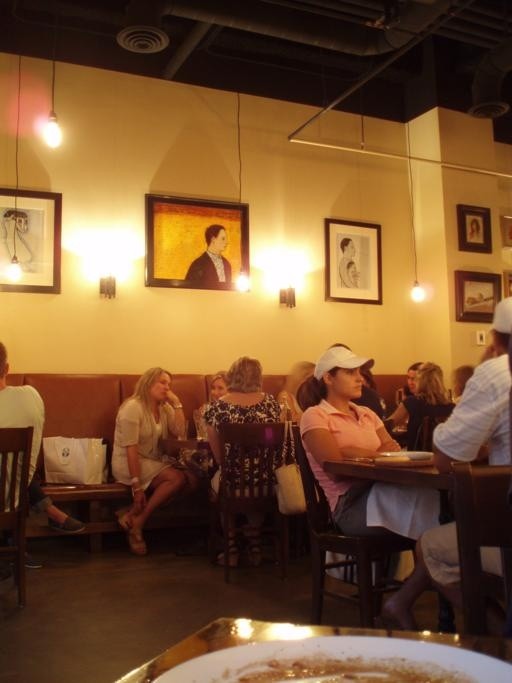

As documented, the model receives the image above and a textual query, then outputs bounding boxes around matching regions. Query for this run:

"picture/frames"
[144,192,251,293]
[323,217,383,306]
[0,188,64,296]
[498,215,512,251]
[501,269,512,298]
[455,203,493,254]
[454,270,502,324]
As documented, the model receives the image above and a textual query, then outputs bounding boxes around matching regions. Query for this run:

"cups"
[177,420,189,441]
[193,410,207,439]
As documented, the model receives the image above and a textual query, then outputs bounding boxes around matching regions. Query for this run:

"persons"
[418,296,512,635]
[337,237,359,289]
[111,367,199,555]
[202,355,280,567]
[23,482,86,569]
[299,347,453,632]
[185,224,232,285]
[0,342,45,511]
[469,219,482,243]
[276,343,473,451]
[194,369,226,441]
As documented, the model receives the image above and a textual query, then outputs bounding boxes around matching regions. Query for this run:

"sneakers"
[47,514,88,535]
[9,556,48,570]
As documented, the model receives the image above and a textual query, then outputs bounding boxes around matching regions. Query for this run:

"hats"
[489,294,512,335]
[312,345,376,381]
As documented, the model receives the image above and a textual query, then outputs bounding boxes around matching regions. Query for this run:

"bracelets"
[129,488,143,495]
[173,403,183,409]
[130,476,139,484]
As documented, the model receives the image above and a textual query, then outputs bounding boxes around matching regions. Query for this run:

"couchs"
[0,372,429,556]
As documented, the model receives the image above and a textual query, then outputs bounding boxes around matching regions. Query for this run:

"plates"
[147,634,512,682]
[380,451,433,460]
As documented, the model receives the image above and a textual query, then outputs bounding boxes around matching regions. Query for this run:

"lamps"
[89,247,123,301]
[38,54,65,150]
[230,89,252,293]
[405,117,427,307]
[4,52,25,286]
[273,266,305,310]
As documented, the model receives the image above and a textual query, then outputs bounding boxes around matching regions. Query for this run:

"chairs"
[206,421,291,587]
[421,415,448,452]
[0,426,35,610]
[291,419,415,628]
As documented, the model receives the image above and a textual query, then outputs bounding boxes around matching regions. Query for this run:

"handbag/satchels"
[41,435,109,485]
[274,462,322,517]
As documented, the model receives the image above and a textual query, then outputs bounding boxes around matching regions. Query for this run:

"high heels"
[213,543,241,567]
[128,527,148,555]
[114,505,134,530]
[372,614,433,632]
[244,545,263,569]
